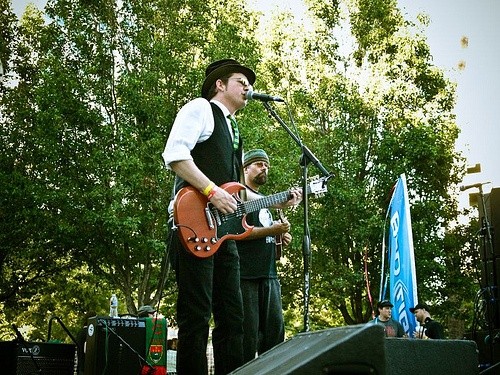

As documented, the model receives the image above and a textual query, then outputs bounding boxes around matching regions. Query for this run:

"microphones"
[460,182,490,190]
[246,91,285,102]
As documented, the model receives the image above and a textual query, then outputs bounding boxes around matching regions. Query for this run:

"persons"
[367,300,405,337]
[409,303,436,339]
[243,149,292,367]
[167,322,179,350]
[162,59,303,375]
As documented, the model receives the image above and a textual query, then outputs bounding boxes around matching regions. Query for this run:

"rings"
[298,197,301,199]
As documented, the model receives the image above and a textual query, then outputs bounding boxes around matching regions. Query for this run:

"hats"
[243,149,269,168]
[378,300,393,308]
[201,59,256,98]
[409,304,430,314]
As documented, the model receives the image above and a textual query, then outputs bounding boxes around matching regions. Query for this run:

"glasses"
[252,162,269,169]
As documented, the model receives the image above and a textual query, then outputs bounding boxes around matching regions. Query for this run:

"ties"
[227,114,239,153]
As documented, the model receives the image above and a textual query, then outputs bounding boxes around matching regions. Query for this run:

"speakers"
[84,316,167,375]
[228,187,500,375]
[0,340,76,375]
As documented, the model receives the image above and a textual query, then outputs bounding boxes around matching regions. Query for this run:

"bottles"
[109,293,118,318]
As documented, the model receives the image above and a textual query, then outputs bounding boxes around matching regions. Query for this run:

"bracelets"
[202,182,218,200]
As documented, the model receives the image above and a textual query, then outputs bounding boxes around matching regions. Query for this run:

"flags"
[390,174,418,338]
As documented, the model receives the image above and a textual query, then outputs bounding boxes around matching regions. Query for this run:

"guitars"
[274,208,285,259]
[173,173,329,259]
